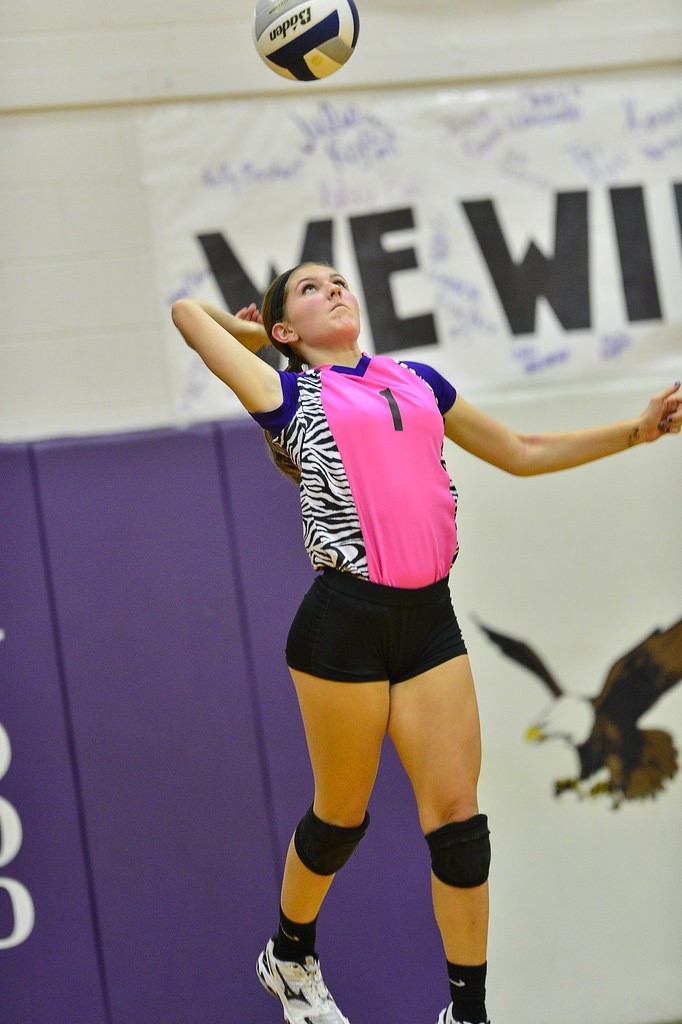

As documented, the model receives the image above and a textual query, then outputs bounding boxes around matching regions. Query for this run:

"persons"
[169,257,681,1024]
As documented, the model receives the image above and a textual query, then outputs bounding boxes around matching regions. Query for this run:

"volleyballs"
[252,1,359,81]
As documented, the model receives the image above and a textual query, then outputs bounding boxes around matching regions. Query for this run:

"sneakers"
[254,934,351,1024]
[434,1000,491,1024]
